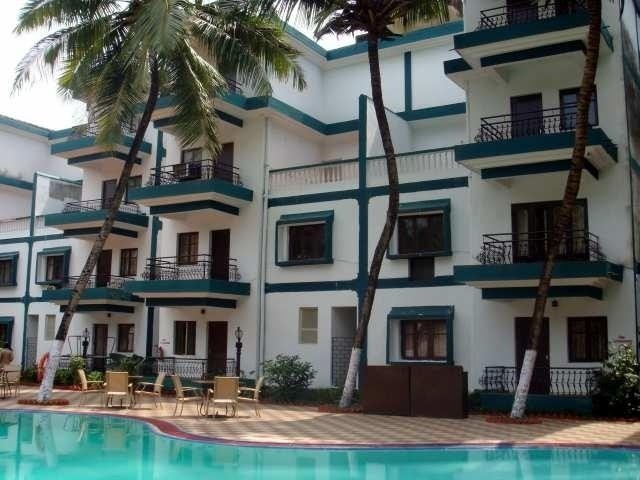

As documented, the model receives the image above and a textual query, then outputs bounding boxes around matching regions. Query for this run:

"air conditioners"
[173,163,192,179]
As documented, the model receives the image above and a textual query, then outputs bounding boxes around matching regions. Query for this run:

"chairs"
[0,364,22,398]
[77,368,168,410]
[171,372,265,420]
